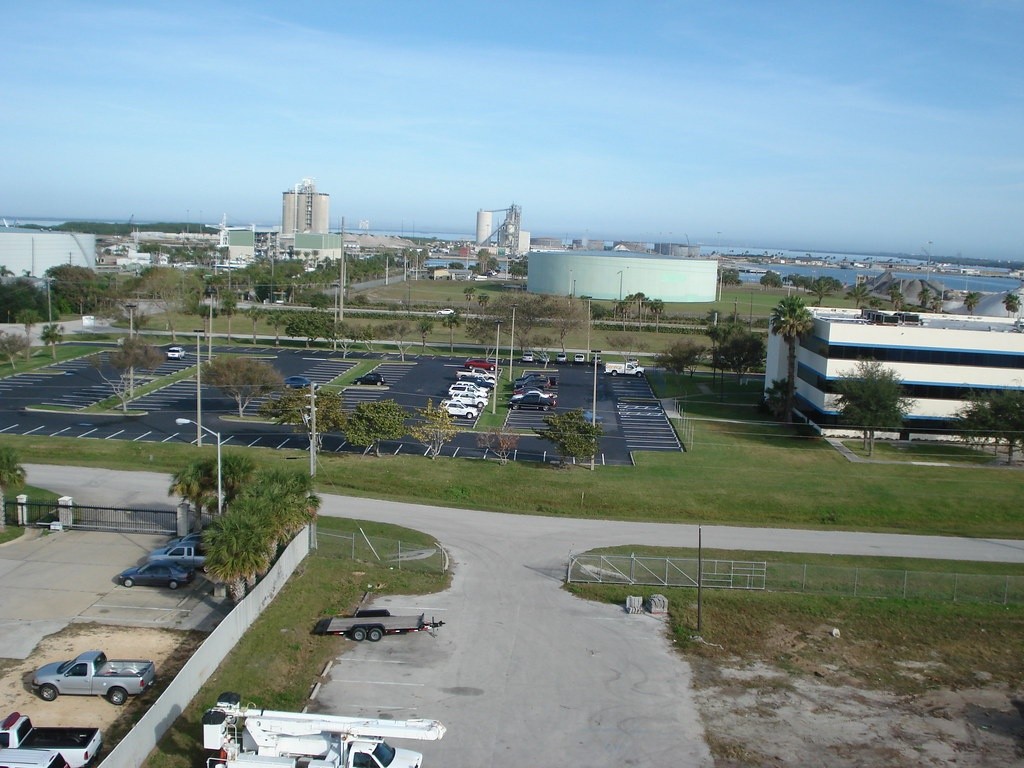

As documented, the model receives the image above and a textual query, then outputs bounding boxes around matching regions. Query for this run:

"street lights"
[176,418,222,515]
[194,330,205,447]
[509,304,519,381]
[586,296,592,362]
[590,350,602,470]
[207,291,216,360]
[126,304,136,397]
[492,319,503,414]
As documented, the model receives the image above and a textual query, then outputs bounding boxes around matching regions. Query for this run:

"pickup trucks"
[0,712,103,768]
[31,651,155,705]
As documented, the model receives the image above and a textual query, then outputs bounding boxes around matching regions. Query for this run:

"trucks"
[604,362,646,378]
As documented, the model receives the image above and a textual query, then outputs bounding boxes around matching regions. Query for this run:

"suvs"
[353,373,385,386]
[0,748,71,768]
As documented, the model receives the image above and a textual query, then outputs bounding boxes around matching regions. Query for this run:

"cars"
[284,376,319,390]
[438,358,495,420]
[507,374,556,410]
[555,352,568,364]
[118,531,207,590]
[167,346,185,360]
[573,353,585,363]
[437,308,456,315]
[591,356,602,366]
[540,354,549,362]
[521,352,536,363]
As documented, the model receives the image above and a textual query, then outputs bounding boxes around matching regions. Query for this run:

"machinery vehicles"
[202,691,447,768]
